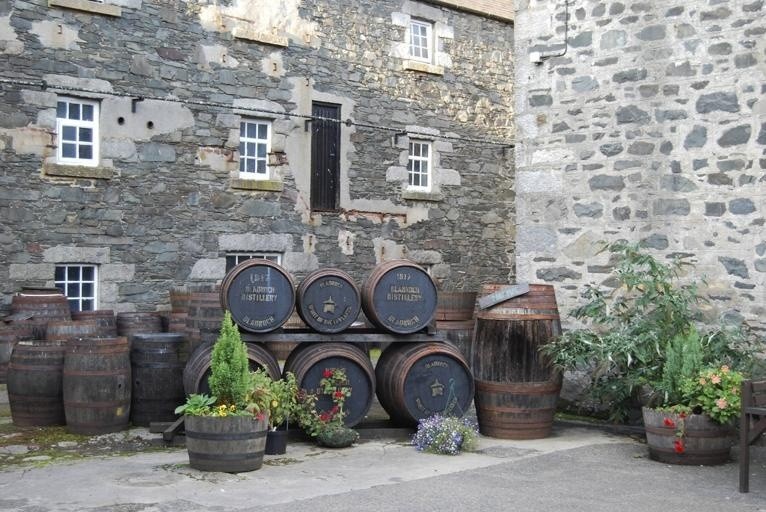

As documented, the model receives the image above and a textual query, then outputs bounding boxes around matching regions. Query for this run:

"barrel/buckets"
[375,340,475,426]
[283,342,375,428]
[182,340,282,402]
[359,257,440,335]
[73,309,116,334]
[131,331,187,428]
[63,334,132,435]
[116,311,164,348]
[6,339,64,427]
[44,319,97,341]
[433,318,476,367]
[296,267,361,334]
[470,283,565,440]
[11,293,73,338]
[219,258,297,333]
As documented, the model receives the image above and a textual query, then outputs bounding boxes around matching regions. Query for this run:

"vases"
[640,405,734,466]
[183,413,268,472]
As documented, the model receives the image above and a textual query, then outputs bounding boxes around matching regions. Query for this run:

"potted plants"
[267,371,298,455]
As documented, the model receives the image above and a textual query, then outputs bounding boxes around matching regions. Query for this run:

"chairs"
[741,379,765,495]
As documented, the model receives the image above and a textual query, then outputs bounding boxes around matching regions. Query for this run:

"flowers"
[296,367,351,438]
[655,365,744,455]
[172,310,282,421]
[411,411,480,455]
